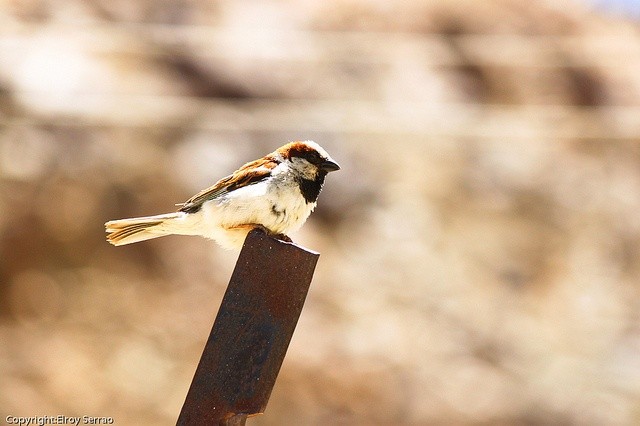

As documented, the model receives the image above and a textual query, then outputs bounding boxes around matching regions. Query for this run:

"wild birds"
[105,140,342,248]
